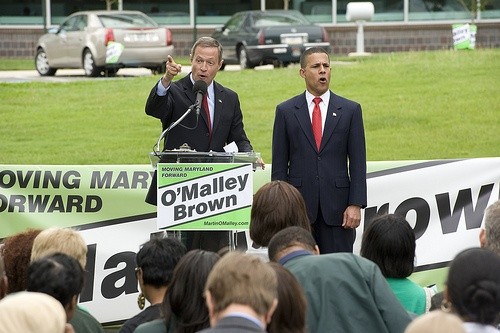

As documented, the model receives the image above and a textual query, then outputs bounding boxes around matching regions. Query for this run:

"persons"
[0,227,104,333]
[144,37,265,251]
[270,47,367,255]
[118,181,500,333]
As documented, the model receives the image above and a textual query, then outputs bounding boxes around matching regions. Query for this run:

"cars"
[33,10,176,78]
[212,10,331,70]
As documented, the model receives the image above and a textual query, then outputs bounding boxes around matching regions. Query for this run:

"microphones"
[193,80,207,115]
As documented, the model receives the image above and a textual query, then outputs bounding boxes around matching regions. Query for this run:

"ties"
[202,90,212,138]
[312,98,322,151]
